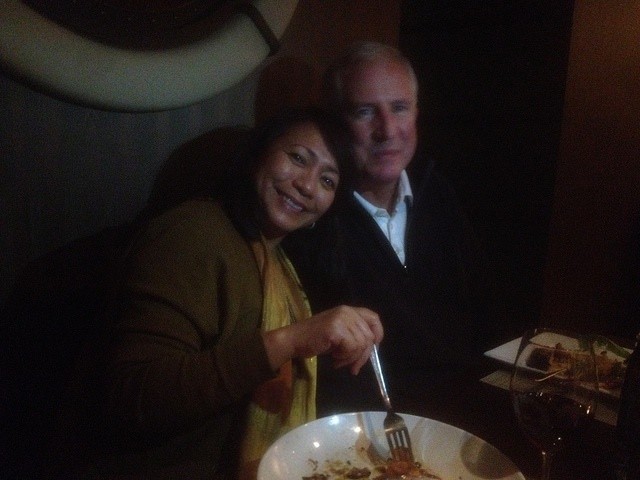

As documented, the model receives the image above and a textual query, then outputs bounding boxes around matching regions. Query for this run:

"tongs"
[586,332,634,390]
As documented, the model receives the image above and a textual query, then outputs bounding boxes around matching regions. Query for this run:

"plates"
[254,410,527,480]
[481,330,635,398]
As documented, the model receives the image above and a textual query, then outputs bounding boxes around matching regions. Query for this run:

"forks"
[368,344,415,466]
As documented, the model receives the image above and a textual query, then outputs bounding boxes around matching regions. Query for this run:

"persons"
[76,104,385,480]
[280,43,488,417]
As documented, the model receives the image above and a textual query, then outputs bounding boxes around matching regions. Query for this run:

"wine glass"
[510,331,597,480]
